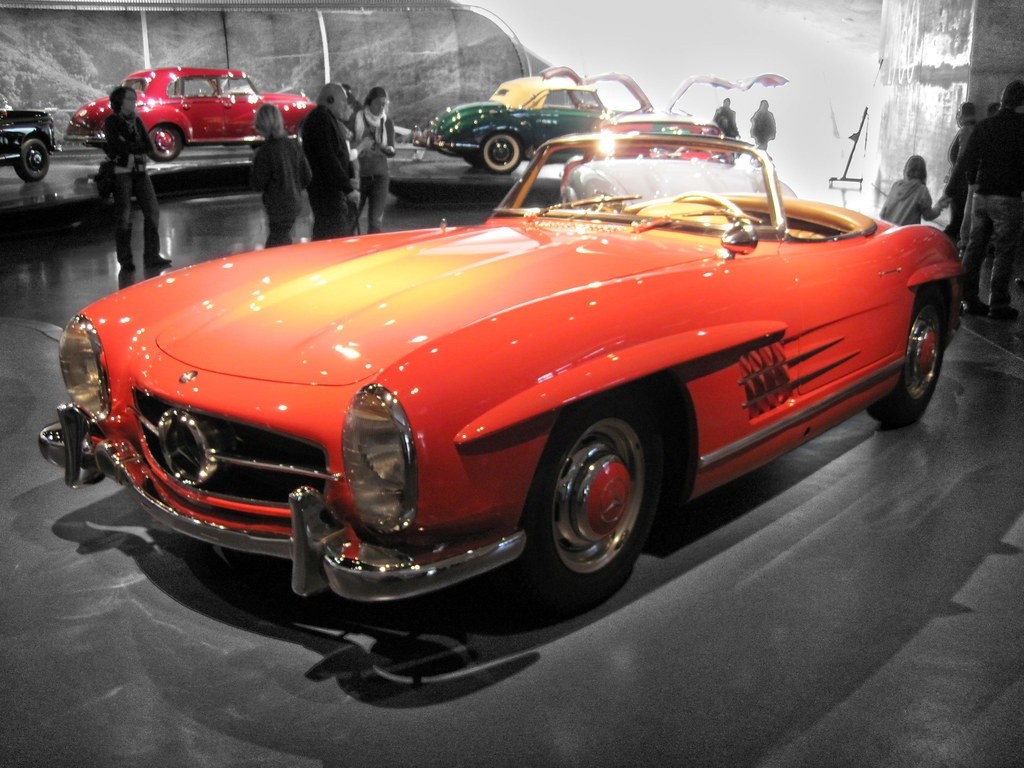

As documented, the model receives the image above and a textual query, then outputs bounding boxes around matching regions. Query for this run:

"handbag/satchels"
[93,161,115,198]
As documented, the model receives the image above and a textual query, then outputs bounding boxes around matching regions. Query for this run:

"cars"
[38,129,969,628]
[0,108,63,183]
[485,111,799,243]
[410,75,661,175]
[63,65,319,164]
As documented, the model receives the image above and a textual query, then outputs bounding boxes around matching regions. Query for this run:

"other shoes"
[119,264,134,278]
[144,257,172,268]
[1015,277,1024,291]
[987,305,1018,320]
[961,303,989,316]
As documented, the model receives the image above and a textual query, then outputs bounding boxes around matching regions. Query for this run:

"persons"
[880,156,943,226]
[942,81,1024,317]
[749,100,776,148]
[302,83,395,241]
[714,98,738,138]
[104,87,172,277]
[252,104,312,250]
